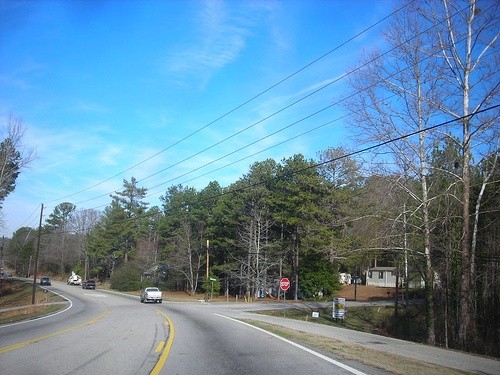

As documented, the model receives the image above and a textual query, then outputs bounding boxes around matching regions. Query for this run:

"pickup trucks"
[141,287,162,304]
[66,275,82,285]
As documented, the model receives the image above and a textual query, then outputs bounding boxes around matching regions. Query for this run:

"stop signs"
[280,278,290,290]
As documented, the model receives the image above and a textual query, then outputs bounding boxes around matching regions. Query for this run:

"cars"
[40,278,50,285]
[81,280,95,289]
